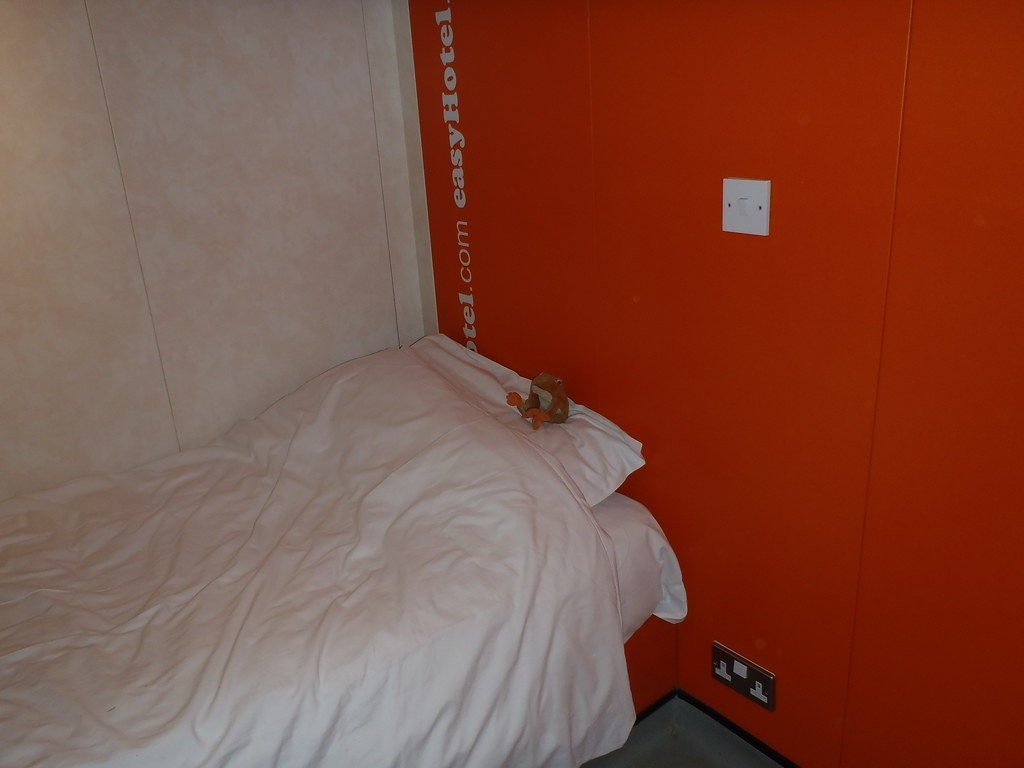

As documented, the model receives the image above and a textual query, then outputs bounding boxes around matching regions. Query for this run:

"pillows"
[384,335,645,510]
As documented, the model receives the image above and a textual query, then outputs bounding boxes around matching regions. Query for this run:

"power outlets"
[710,640,775,714]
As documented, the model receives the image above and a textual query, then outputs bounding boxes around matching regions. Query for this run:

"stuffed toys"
[507,370,570,429]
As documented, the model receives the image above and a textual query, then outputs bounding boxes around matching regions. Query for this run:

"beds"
[0,333,691,767]
